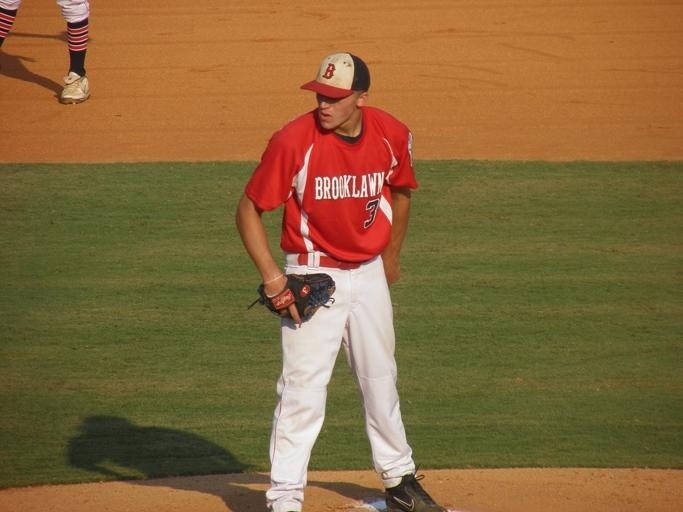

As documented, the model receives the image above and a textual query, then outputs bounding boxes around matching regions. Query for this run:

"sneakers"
[59,72,90,105]
[381,475,446,511]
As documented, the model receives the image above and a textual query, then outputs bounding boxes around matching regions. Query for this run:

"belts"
[296,250,364,272]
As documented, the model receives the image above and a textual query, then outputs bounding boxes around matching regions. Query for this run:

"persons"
[0,1,90,104]
[237,51,447,511]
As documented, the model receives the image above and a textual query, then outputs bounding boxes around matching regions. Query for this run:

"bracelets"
[262,272,282,285]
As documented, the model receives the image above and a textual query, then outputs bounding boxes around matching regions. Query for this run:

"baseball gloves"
[257,274,336,321]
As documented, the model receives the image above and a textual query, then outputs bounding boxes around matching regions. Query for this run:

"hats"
[298,52,370,101]
